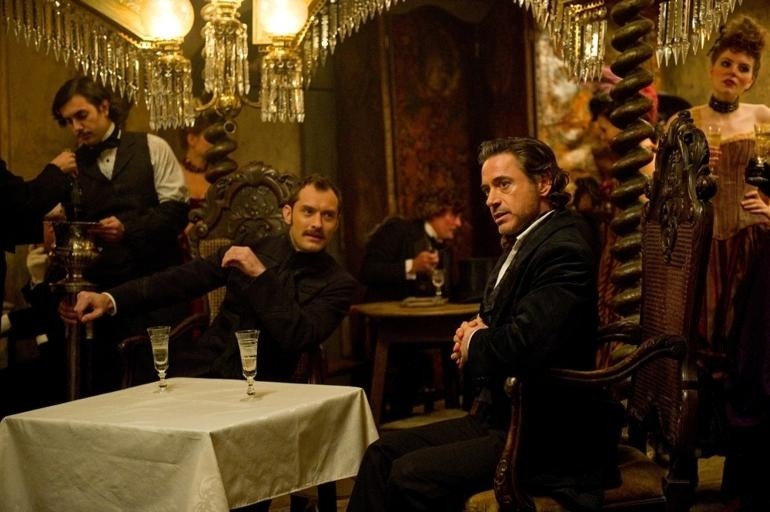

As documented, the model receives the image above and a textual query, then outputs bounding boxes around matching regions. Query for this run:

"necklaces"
[184,160,208,175]
[710,93,739,114]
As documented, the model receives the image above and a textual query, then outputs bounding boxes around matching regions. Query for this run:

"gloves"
[26,244,48,283]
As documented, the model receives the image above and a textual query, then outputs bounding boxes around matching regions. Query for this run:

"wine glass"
[146,325,171,396]
[432,269,444,299]
[704,121,722,175]
[234,329,261,404]
[752,123,770,196]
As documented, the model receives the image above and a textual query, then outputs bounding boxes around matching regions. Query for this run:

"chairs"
[470,110,715,512]
[119,160,327,387]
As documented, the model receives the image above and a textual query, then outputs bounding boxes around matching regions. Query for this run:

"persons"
[0,149,81,322]
[588,84,659,179]
[665,15,770,377]
[175,107,215,336]
[0,203,75,335]
[51,75,191,385]
[349,185,468,413]
[346,135,624,512]
[59,173,356,393]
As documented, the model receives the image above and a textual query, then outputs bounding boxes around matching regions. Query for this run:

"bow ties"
[93,138,121,154]
[429,234,444,252]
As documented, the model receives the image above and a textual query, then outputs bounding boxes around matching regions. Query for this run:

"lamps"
[1,0,408,131]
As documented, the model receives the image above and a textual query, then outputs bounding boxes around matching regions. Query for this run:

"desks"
[0,376,381,511]
[350,298,483,425]
